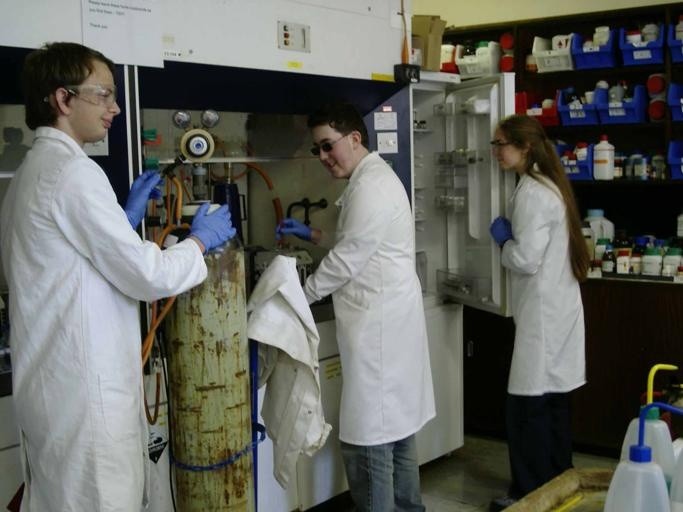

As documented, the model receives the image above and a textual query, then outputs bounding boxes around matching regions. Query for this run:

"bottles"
[582,221,683,276]
[609,79,628,102]
[604,403,683,512]
[648,72,667,121]
[461,40,488,59]
[567,134,667,181]
[583,15,683,48]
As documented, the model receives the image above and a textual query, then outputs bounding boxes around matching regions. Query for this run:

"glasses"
[311,134,348,155]
[44,85,118,106]
[490,139,511,145]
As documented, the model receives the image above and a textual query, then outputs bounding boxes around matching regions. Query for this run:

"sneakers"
[491,497,514,506]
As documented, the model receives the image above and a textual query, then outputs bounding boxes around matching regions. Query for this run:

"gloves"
[489,216,513,247]
[125,169,164,231]
[190,203,237,255]
[275,218,312,241]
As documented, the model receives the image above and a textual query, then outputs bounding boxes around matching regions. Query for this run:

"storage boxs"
[411,14,447,71]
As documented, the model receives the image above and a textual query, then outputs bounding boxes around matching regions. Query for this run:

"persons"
[1,42,238,512]
[288,101,436,512]
[489,113,589,509]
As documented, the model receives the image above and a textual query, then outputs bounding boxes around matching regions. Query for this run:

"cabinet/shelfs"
[439,0,683,456]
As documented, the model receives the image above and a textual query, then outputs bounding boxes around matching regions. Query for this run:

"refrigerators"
[410,71,518,466]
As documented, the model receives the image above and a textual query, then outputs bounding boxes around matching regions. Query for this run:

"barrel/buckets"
[602,444,671,512]
[584,209,614,245]
[668,438,682,512]
[618,405,673,496]
